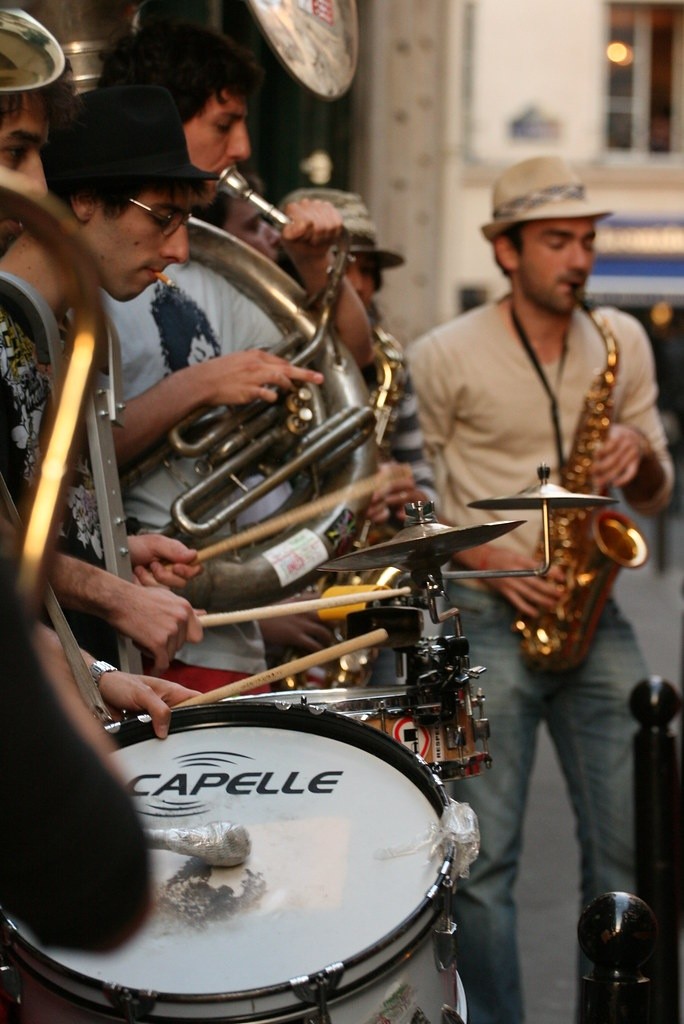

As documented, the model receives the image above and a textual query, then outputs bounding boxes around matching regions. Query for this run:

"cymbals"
[466,481,620,510]
[314,518,526,585]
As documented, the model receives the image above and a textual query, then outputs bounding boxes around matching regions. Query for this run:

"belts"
[448,562,493,593]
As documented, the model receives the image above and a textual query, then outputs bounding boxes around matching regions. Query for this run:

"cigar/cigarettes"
[155,272,176,288]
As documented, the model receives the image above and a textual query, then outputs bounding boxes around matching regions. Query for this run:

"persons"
[405,155,674,1024]
[0,575,154,955]
[0,22,441,755]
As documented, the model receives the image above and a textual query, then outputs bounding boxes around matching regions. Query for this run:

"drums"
[4,699,471,1024]
[226,686,493,778]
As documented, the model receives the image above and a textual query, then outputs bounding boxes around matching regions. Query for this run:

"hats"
[481,155,616,243]
[39,84,221,190]
[273,188,406,269]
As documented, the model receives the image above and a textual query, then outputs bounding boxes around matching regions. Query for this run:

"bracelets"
[90,661,118,686]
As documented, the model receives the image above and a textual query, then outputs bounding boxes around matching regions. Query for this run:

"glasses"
[116,192,194,238]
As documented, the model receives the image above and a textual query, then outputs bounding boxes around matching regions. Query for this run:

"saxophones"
[364,306,415,443]
[512,285,652,680]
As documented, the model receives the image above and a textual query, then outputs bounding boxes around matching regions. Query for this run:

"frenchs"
[50,2,384,614]
